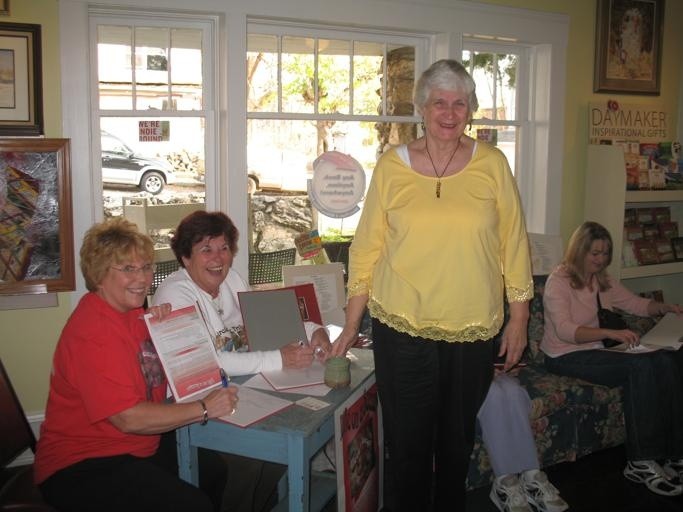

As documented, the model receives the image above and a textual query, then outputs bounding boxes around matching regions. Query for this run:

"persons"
[539,220,683,498]
[147,209,332,512]
[34,216,242,512]
[476,336,572,511]
[315,60,537,511]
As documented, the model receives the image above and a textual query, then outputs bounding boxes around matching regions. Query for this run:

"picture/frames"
[591,1,663,97]
[0,21,77,297]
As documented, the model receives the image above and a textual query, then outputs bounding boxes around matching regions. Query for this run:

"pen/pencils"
[494,363,527,366]
[220,368,228,387]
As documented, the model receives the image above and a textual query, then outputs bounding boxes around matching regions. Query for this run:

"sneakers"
[489,475,534,512]
[662,459,683,479]
[623,459,682,496]
[518,470,570,511]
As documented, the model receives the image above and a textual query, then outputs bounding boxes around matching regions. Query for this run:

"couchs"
[452,276,664,494]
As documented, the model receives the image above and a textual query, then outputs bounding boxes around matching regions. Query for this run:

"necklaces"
[421,132,467,202]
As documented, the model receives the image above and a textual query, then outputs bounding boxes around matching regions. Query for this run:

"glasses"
[110,262,157,276]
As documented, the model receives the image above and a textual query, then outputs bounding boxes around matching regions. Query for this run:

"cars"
[99,130,178,195]
[180,140,312,195]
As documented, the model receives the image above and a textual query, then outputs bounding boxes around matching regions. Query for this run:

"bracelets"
[195,399,209,425]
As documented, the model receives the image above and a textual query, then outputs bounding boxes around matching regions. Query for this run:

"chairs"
[0,358,56,512]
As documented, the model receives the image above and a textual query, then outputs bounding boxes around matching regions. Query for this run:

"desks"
[173,347,379,512]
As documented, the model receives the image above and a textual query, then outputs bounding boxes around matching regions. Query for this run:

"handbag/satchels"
[596,290,630,348]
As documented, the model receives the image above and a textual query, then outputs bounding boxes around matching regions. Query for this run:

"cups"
[324,357,351,388]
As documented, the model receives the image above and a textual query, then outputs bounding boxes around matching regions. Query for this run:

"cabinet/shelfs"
[585,144,683,281]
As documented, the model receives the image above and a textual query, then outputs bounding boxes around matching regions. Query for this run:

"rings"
[230,407,236,414]
